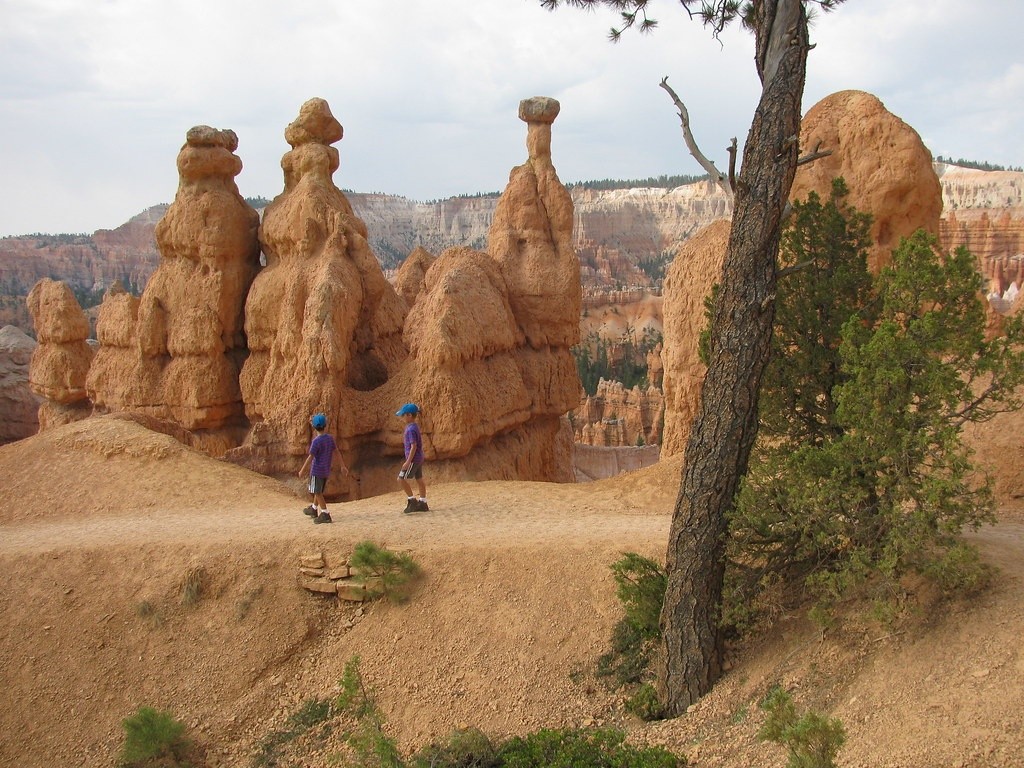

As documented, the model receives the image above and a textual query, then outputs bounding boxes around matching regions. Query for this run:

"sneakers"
[416,500,429,511]
[303,505,318,518]
[314,512,332,524]
[404,498,418,513]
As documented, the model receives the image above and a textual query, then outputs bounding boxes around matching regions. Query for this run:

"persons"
[298,414,349,524]
[396,403,429,512]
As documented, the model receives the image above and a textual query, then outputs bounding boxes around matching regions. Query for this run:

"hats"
[313,414,326,428]
[395,404,418,415]
